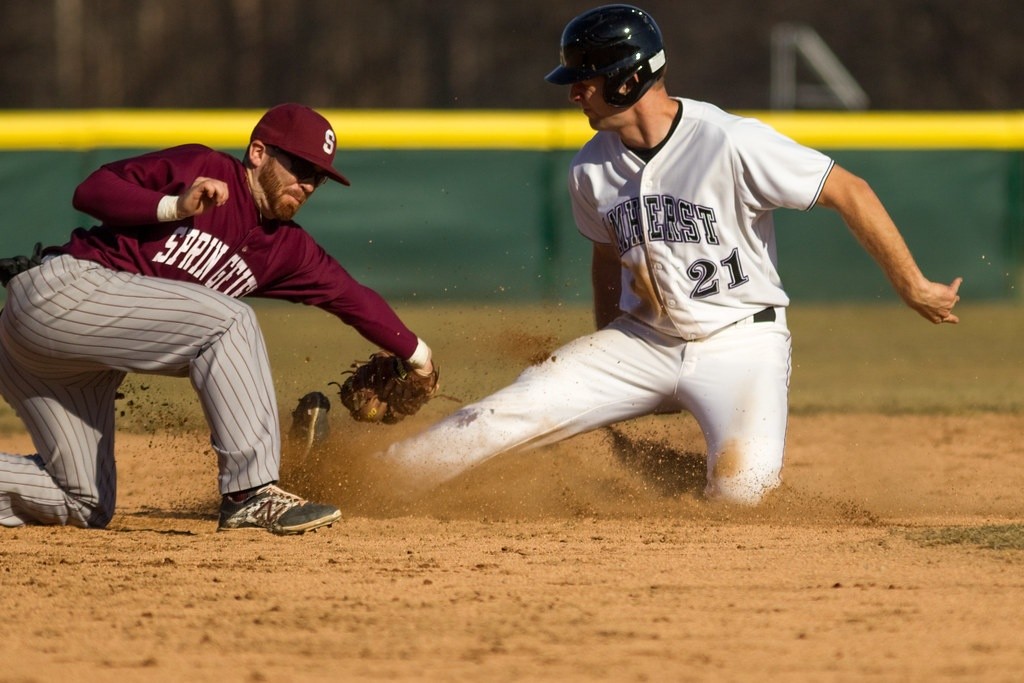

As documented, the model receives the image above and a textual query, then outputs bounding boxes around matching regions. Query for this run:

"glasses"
[272,146,330,185]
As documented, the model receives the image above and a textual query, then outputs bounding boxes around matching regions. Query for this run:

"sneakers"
[216,483,343,533]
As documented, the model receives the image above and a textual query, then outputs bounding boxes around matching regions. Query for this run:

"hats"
[251,102,351,187]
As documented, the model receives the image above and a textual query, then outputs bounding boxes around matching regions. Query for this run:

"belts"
[754,307,776,323]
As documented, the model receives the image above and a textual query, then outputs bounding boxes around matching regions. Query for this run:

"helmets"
[543,4,668,106]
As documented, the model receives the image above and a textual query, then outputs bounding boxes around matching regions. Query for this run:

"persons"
[289,4,963,509]
[0,102,440,530]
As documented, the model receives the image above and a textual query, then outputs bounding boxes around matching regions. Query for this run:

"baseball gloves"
[326,350,443,426]
